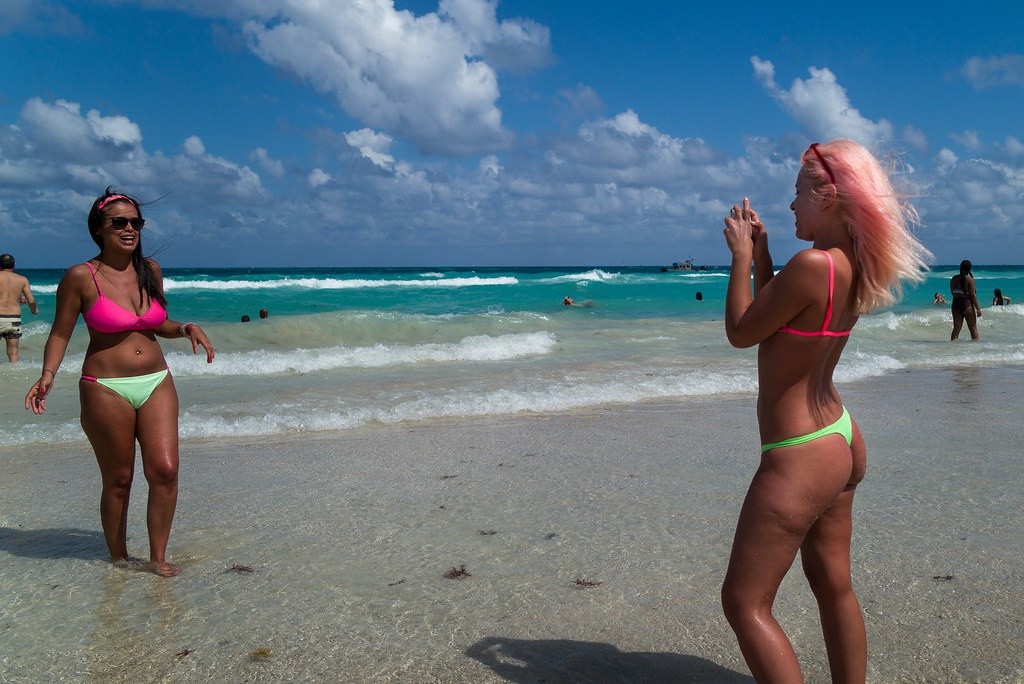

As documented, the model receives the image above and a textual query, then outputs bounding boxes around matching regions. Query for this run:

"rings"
[211,346,214,350]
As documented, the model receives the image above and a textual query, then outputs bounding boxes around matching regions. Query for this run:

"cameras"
[731,209,752,223]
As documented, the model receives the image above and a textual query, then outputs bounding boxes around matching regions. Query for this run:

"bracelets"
[42,369,55,380]
[180,322,197,340]
[977,309,980,310]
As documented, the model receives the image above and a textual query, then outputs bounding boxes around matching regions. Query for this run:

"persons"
[950,260,983,340]
[993,288,1011,306]
[564,297,574,305]
[23,185,216,577]
[720,138,936,684]
[0,254,39,363]
[241,315,250,322]
[933,293,947,304]
[260,308,269,319]
[696,292,702,300]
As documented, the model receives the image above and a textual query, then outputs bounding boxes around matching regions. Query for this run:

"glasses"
[803,143,835,184]
[98,217,145,231]
